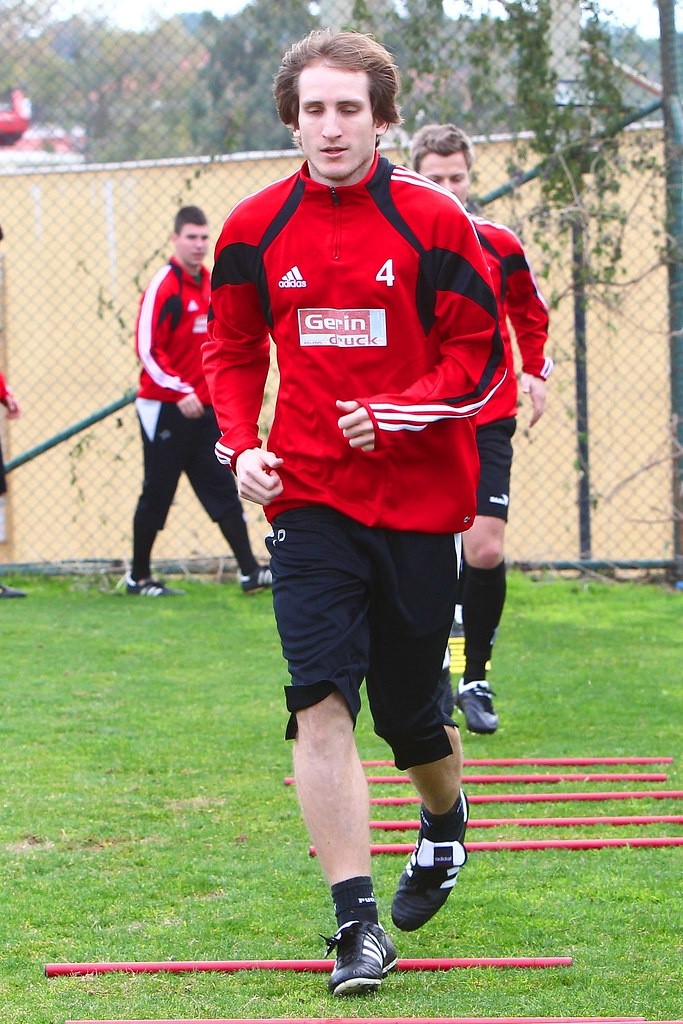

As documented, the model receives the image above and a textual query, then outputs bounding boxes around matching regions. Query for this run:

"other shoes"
[0,582,26,597]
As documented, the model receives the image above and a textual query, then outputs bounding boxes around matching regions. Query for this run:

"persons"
[0,224,29,598]
[201,27,508,999]
[124,206,274,598]
[398,123,553,735]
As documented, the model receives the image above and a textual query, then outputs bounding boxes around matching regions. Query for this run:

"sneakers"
[241,565,273,594]
[436,643,454,716]
[319,919,396,994]
[452,675,498,735]
[390,790,469,929]
[125,574,185,596]
